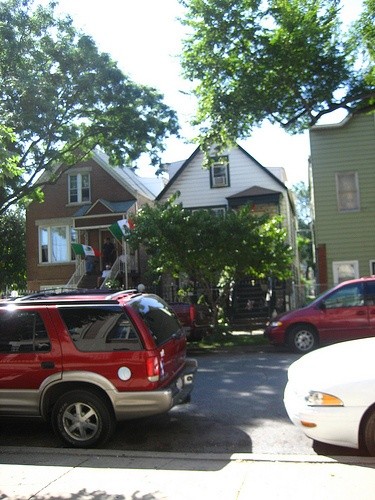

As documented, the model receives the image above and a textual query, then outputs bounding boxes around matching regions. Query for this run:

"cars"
[283,336,375,457]
[265,275,375,352]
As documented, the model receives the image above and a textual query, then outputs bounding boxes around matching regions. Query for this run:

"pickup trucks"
[118,302,207,342]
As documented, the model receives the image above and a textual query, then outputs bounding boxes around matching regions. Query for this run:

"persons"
[102,237,115,269]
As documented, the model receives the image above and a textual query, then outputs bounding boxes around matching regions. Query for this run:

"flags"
[71,243,101,257]
[108,218,135,241]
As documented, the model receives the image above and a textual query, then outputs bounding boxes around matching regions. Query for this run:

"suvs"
[0,285,200,451]
